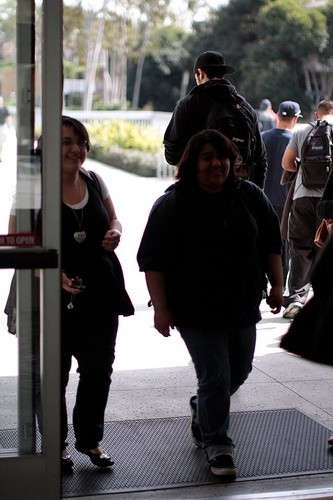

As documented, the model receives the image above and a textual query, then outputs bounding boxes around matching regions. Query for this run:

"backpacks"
[301,120,333,191]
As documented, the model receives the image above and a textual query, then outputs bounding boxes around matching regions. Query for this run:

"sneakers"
[189,395,206,448]
[283,302,304,319]
[211,455,237,475]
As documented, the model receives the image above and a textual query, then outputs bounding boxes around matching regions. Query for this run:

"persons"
[135,129,283,477]
[163,51,268,190]
[254,100,333,444]
[4,116,135,469]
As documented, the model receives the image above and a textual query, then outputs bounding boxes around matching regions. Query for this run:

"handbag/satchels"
[90,171,135,317]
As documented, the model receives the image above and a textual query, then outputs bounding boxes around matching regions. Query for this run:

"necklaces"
[62,177,87,243]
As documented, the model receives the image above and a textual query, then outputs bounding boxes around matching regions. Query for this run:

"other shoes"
[61,454,74,469]
[75,445,114,467]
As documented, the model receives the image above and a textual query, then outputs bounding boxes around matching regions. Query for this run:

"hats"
[279,101,301,117]
[193,51,235,73]
